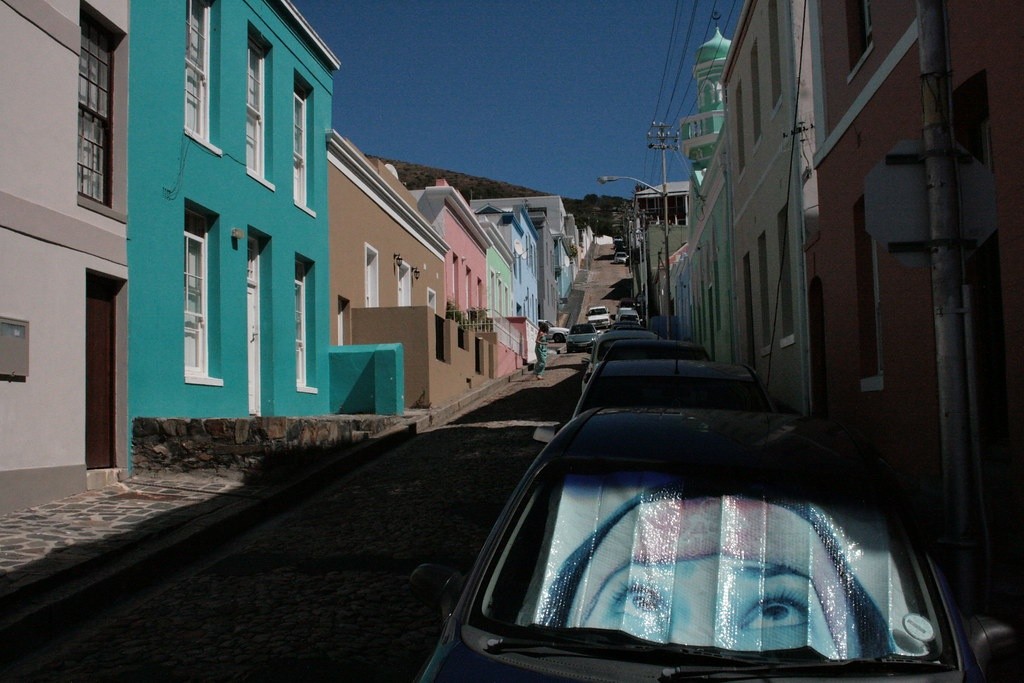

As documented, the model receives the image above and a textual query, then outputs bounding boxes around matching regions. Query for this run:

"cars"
[412,307,986,683]
[614,252,629,264]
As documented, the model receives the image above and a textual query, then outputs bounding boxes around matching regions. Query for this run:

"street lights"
[597,176,672,341]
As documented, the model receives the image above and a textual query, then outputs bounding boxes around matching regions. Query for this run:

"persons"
[531,323,549,380]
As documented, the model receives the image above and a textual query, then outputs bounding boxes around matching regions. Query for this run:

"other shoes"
[536,374,543,380]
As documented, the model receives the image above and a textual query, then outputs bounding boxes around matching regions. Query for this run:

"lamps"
[412,266,420,281]
[232,229,243,239]
[393,253,402,267]
